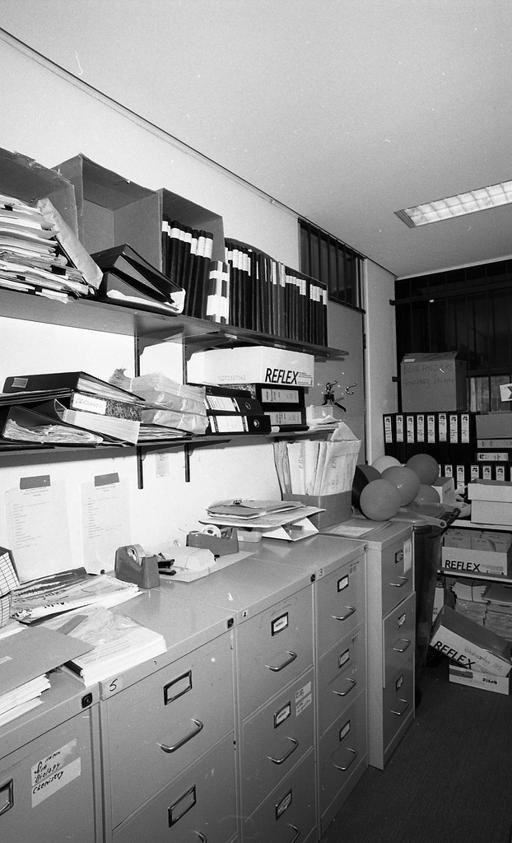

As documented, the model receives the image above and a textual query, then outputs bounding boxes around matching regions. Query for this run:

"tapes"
[201,524,221,538]
[127,544,146,566]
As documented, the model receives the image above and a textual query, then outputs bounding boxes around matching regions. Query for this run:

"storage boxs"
[401,350,512,695]
[155,188,226,262]
[51,154,162,274]
[281,491,352,530]
[0,148,79,239]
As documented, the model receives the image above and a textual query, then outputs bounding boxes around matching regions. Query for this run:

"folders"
[3,372,146,406]
[383,411,512,504]
[89,243,183,316]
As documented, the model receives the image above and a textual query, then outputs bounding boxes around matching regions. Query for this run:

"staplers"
[158,559,176,575]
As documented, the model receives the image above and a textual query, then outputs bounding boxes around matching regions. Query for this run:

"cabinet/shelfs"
[320,516,415,775]
[0,533,366,843]
[0,288,348,468]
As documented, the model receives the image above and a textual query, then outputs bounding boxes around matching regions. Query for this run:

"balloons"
[358,454,442,522]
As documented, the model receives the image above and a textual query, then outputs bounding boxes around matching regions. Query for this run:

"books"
[1,195,104,304]
[272,433,363,495]
[224,237,328,347]
[382,373,512,504]
[162,215,228,324]
[452,578,511,642]
[0,565,168,728]
[2,369,209,453]
[187,383,310,435]
